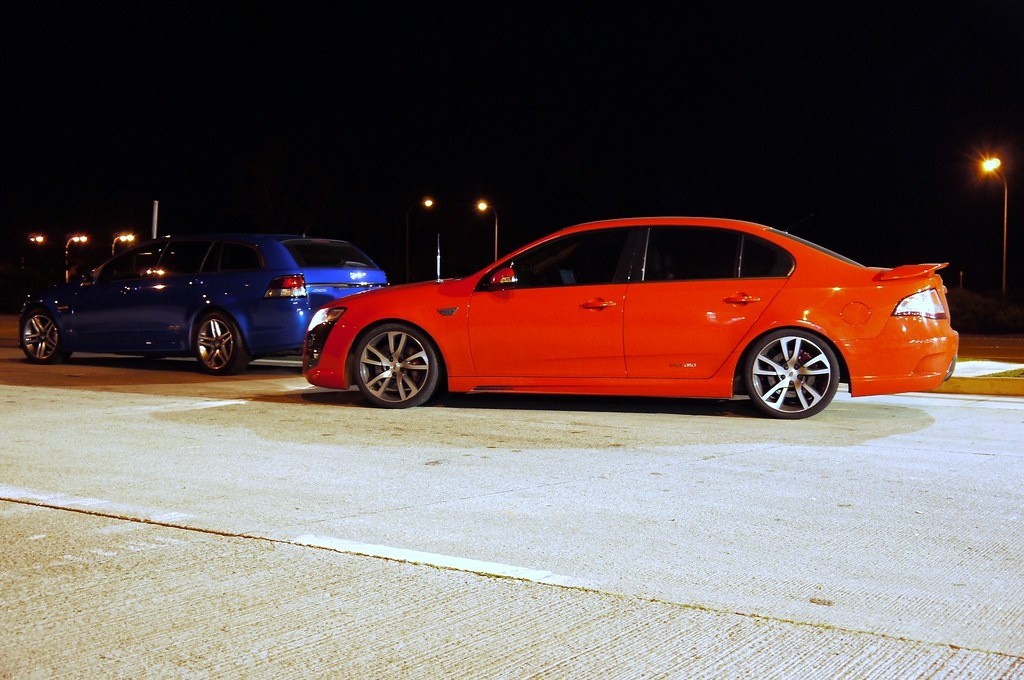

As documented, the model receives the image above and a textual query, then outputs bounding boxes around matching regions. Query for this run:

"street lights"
[478,203,498,263]
[65,236,87,284]
[424,200,442,280]
[112,235,135,257]
[981,157,1008,296]
[21,236,43,299]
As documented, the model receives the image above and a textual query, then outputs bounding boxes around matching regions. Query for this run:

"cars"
[19,235,391,376]
[302,217,960,420]
